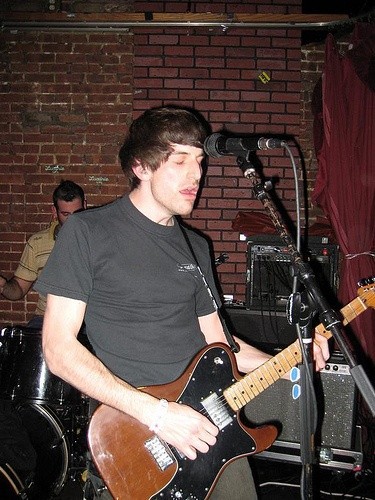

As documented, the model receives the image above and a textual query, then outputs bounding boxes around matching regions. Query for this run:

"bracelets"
[147,397,169,431]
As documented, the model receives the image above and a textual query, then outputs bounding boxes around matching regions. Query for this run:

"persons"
[33,105,332,500]
[1,180,85,316]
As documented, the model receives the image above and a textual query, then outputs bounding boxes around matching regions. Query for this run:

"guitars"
[85,276,375,500]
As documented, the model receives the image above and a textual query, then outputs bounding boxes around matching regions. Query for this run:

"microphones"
[203,132,286,161]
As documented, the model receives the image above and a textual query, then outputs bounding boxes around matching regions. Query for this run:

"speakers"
[223,303,311,356]
[236,359,362,456]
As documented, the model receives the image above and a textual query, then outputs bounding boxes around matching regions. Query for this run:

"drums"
[0,402,81,500]
[0,326,76,402]
